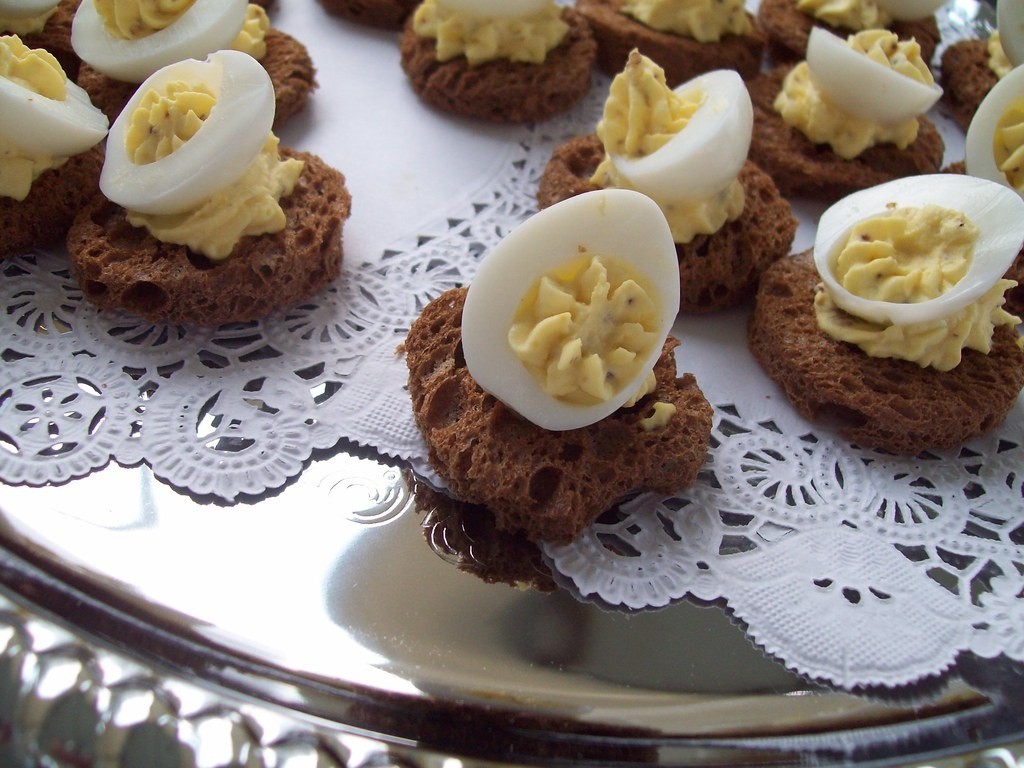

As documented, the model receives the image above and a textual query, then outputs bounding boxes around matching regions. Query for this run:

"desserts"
[0,0,1024,543]
